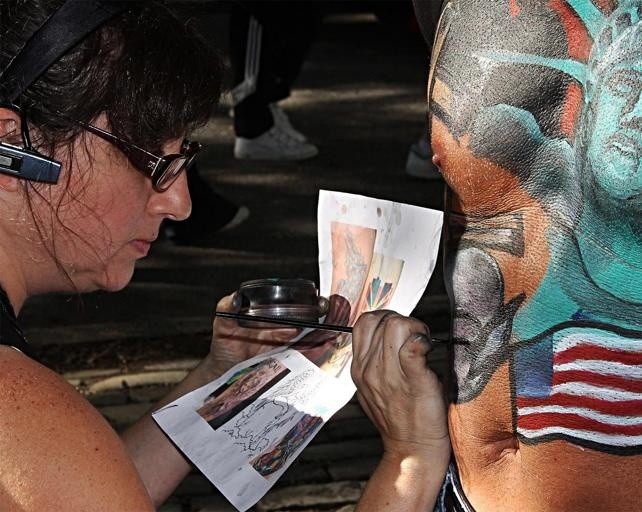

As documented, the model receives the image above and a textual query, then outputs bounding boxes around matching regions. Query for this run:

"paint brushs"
[214,311,448,346]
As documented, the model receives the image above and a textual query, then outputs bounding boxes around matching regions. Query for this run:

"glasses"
[17,92,206,195]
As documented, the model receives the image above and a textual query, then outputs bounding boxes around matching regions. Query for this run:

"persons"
[427,1,640,510]
[226,1,322,162]
[394,1,450,181]
[1,1,450,511]
[470,2,640,451]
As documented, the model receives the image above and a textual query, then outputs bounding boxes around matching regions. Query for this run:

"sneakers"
[268,101,311,144]
[404,141,446,182]
[232,124,321,163]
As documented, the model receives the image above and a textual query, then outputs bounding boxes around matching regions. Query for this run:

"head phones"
[0,142,62,186]
